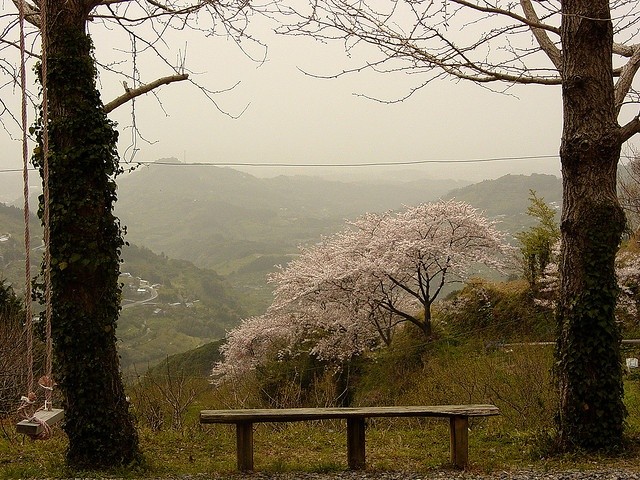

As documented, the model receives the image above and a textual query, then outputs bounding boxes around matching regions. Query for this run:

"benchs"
[200,404,498,469]
